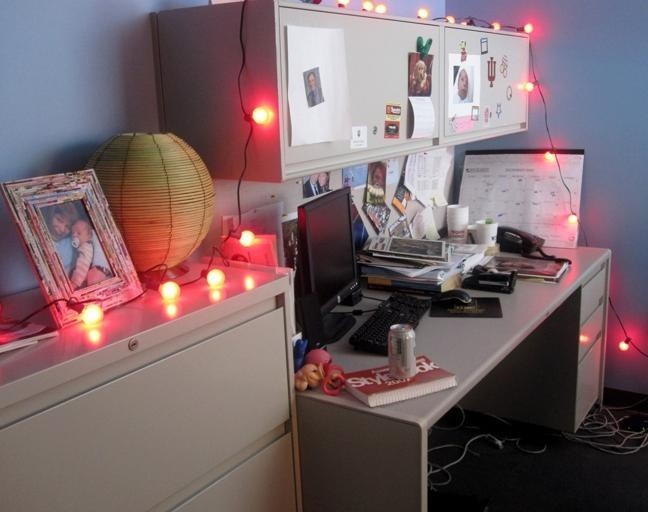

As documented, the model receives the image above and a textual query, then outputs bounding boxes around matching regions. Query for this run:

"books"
[0,319,59,350]
[0,339,39,355]
[353,233,572,296]
[339,353,459,407]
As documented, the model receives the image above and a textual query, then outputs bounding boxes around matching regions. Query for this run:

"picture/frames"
[2,168,144,332]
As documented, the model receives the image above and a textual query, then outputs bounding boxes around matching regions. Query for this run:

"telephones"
[497,226,545,254]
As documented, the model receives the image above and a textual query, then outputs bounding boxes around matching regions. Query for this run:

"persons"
[66,219,104,288]
[48,201,111,287]
[305,73,323,106]
[317,173,331,195]
[401,191,411,208]
[450,65,476,104]
[303,174,320,198]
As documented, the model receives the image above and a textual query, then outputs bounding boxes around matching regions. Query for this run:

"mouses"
[437,289,473,305]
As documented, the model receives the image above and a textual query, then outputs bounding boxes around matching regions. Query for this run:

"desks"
[294,243,613,512]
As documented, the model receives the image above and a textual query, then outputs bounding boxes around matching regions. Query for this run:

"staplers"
[462,274,513,294]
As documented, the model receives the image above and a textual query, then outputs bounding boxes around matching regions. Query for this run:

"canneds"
[388,323,416,379]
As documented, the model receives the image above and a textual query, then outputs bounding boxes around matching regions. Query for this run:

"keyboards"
[348,293,430,356]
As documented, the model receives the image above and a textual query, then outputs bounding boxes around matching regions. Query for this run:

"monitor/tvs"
[297,185,361,345]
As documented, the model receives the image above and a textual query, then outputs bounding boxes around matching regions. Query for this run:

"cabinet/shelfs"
[0,259,304,512]
[151,2,531,184]
